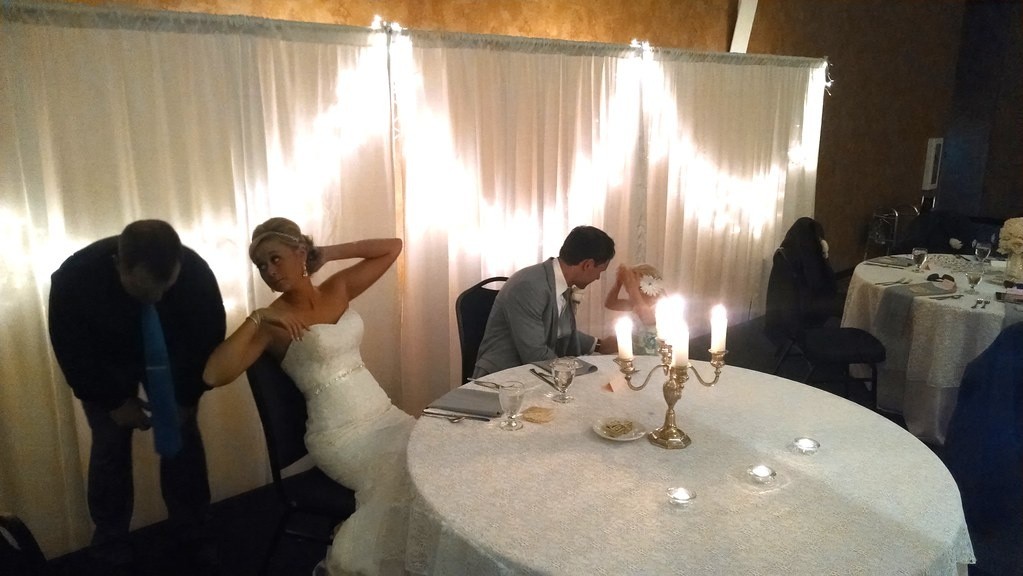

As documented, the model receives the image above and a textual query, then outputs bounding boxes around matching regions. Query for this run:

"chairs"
[454,276,510,388]
[939,319,1023,576]
[767,210,1007,406]
[247,348,357,576]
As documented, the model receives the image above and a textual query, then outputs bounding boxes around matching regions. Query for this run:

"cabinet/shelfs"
[864,204,921,260]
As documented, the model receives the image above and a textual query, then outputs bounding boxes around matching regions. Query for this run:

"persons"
[472,226,640,381]
[47,219,228,576]
[202,218,419,576]
[605,264,666,356]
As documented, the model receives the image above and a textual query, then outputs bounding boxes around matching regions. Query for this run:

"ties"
[561,288,573,357]
[143,303,180,459]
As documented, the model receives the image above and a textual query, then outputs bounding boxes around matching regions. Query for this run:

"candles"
[710,304,727,351]
[616,316,634,360]
[656,294,689,366]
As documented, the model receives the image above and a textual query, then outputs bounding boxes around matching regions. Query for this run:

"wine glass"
[496,381,524,431]
[975,242,992,267]
[966,270,982,295]
[550,358,575,403]
[911,248,928,273]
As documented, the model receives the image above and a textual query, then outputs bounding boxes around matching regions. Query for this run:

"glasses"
[927,274,954,282]
[1004,280,1023,289]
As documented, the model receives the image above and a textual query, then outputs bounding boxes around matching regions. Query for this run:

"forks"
[980,296,991,309]
[876,278,913,286]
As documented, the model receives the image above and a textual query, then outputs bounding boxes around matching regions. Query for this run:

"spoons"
[970,298,983,308]
[421,412,465,423]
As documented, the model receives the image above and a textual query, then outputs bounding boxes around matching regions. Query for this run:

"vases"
[1003,249,1023,289]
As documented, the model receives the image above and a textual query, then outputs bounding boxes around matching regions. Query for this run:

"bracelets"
[595,339,601,352]
[251,310,261,326]
[246,316,258,330]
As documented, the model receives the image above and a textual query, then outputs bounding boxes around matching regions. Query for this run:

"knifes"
[421,410,491,422]
[529,368,566,394]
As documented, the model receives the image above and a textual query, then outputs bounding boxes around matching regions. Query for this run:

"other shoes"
[184,538,224,576]
[112,562,143,576]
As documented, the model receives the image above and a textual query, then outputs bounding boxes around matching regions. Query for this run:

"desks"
[839,252,1023,448]
[401,355,975,576]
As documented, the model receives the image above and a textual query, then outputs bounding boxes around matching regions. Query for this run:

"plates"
[591,416,647,441]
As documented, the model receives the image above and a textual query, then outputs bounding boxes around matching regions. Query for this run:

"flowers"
[639,274,666,298]
[997,216,1023,256]
